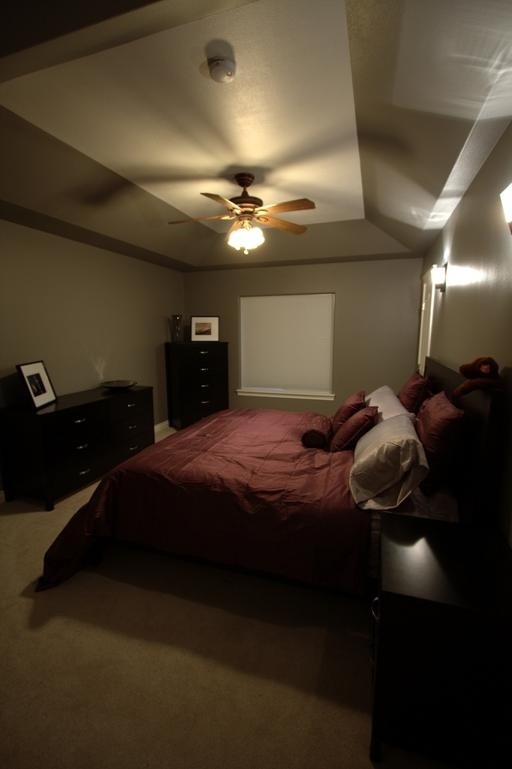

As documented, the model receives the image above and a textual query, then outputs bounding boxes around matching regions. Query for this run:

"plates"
[100,380,135,389]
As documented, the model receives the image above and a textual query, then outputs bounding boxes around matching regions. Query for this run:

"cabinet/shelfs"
[164,341,229,431]
[0,383,155,510]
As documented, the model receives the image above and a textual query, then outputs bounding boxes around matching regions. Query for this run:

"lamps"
[226,218,266,255]
[431,263,447,292]
[499,182,512,239]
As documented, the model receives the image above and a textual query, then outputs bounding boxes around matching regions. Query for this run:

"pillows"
[301,370,464,510]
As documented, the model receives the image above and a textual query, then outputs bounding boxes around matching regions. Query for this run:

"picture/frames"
[16,360,57,409]
[191,316,220,342]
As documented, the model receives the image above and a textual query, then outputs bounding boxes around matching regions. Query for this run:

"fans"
[168,173,315,236]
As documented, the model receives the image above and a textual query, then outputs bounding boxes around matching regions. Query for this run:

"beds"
[38,356,512,590]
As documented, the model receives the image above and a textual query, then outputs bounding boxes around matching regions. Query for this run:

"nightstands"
[368,513,512,769]
